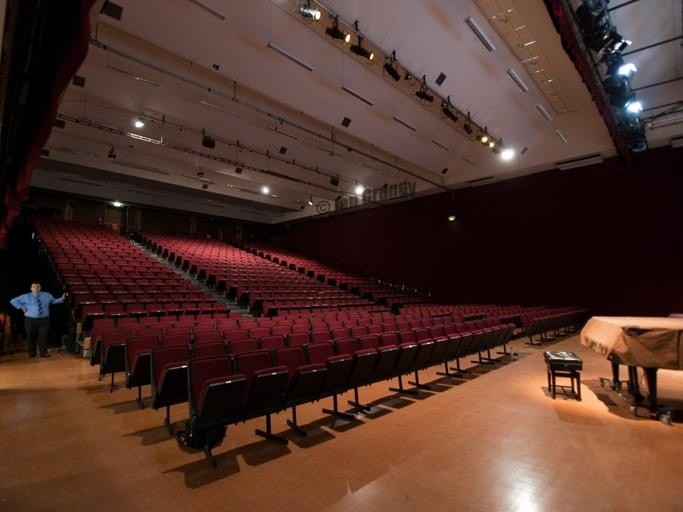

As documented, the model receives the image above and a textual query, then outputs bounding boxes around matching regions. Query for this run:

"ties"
[36,294,43,316]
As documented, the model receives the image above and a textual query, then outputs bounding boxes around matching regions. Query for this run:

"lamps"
[383,49,473,136]
[297,1,374,61]
[575,1,646,150]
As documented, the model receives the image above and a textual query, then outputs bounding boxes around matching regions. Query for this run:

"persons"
[9,282,69,358]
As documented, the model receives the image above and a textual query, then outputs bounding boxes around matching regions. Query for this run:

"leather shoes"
[29,354,35,358]
[40,353,49,357]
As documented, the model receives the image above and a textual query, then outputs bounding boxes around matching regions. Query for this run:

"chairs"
[28,218,590,469]
